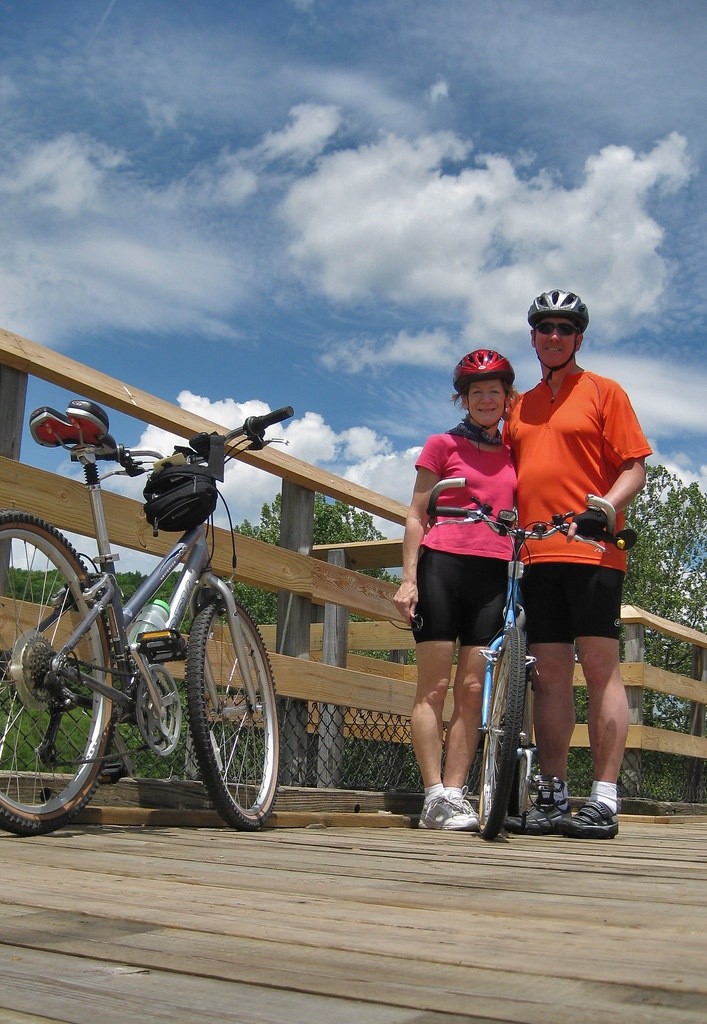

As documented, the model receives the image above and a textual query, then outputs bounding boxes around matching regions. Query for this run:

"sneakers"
[418,796,477,831]
[446,786,478,818]
[555,801,618,839]
[503,797,572,833]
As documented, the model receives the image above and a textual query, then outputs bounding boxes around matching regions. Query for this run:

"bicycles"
[425,478,639,840]
[0,395,297,838]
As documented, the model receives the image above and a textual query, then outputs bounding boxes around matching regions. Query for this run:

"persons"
[500,290,647,840]
[391,348,520,832]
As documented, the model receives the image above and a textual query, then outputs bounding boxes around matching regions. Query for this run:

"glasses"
[389,614,423,631]
[534,322,579,336]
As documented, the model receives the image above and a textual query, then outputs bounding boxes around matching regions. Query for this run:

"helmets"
[528,289,589,334]
[453,350,515,392]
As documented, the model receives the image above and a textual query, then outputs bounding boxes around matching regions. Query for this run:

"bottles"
[128,599,170,661]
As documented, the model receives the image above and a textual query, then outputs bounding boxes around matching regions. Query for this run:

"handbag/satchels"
[143,435,224,537]
